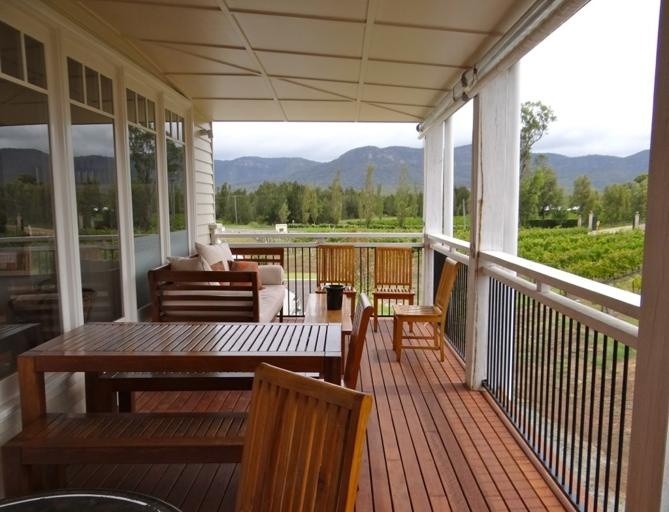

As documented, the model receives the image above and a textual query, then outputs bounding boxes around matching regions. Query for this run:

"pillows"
[166,241,286,291]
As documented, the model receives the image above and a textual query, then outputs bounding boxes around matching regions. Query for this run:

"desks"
[14,320,345,490]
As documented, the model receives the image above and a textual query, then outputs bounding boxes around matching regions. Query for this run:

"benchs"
[98,371,319,413]
[2,412,253,500]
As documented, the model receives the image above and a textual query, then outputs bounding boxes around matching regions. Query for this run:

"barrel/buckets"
[325,285,345,311]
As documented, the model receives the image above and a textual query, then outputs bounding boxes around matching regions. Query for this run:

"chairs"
[315,244,459,362]
[235,291,376,511]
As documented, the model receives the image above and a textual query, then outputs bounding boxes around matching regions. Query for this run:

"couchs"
[146,245,286,323]
